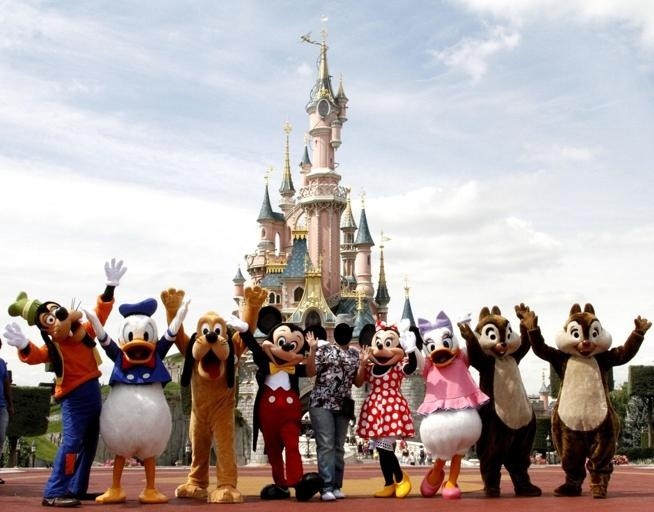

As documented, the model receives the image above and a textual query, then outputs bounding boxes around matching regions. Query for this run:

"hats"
[333,314,355,329]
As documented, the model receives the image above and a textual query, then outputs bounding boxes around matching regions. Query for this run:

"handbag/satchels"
[342,397,355,417]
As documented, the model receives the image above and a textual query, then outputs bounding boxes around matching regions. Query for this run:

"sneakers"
[333,489,346,499]
[322,491,336,501]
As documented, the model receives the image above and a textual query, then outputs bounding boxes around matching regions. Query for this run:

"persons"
[357,438,432,465]
[304,314,373,500]
[0,338,15,485]
